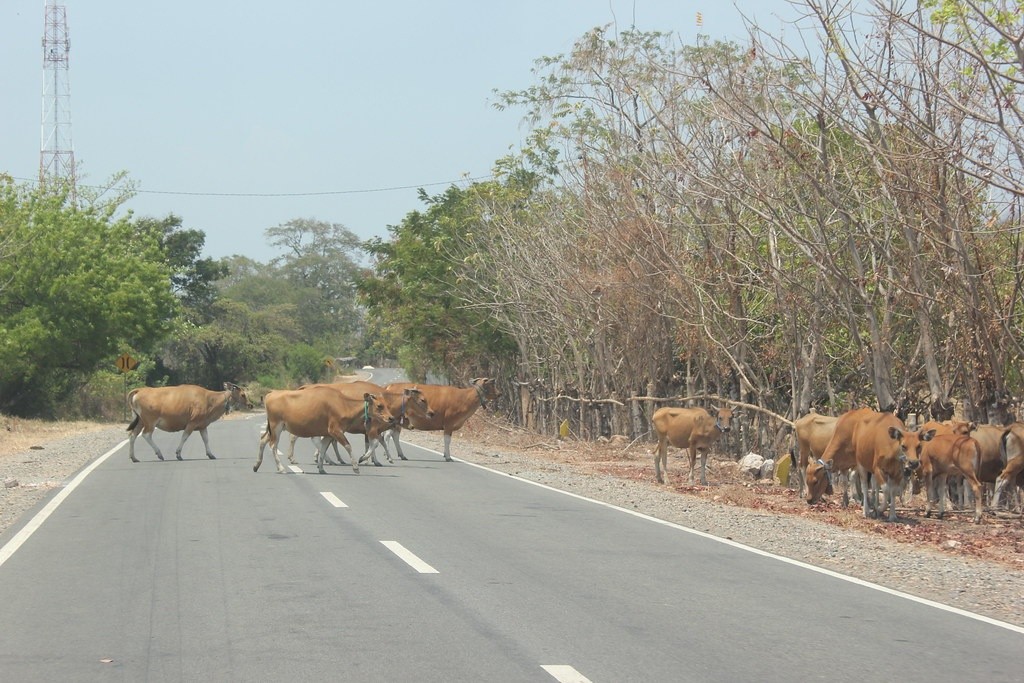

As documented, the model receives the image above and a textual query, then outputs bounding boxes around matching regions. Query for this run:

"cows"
[649,403,738,487]
[125,376,503,474]
[789,407,1024,523]
